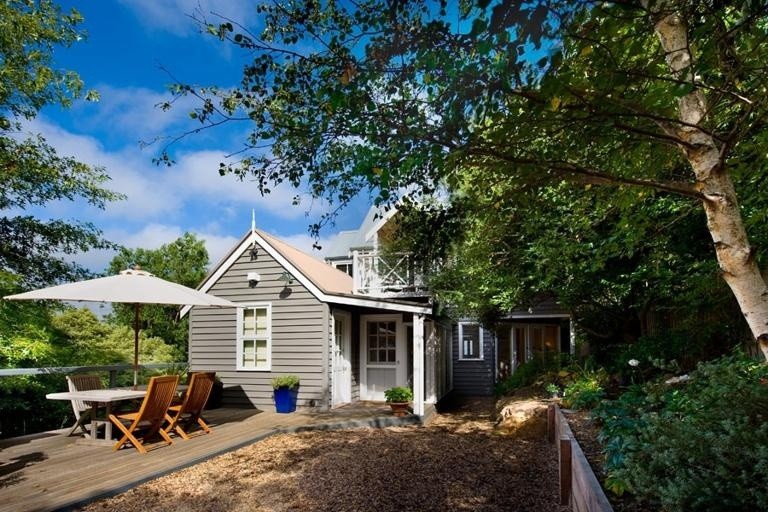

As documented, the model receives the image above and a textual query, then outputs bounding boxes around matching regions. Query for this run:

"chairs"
[163,372,216,440]
[66,375,108,438]
[109,375,180,453]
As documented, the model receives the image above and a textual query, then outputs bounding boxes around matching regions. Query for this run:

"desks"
[47,383,240,446]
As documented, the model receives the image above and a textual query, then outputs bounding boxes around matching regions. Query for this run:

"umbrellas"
[2,264,245,391]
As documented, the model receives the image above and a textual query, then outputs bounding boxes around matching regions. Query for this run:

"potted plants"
[385,386,413,417]
[272,376,300,413]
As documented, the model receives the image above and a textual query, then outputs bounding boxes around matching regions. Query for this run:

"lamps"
[276,272,293,285]
